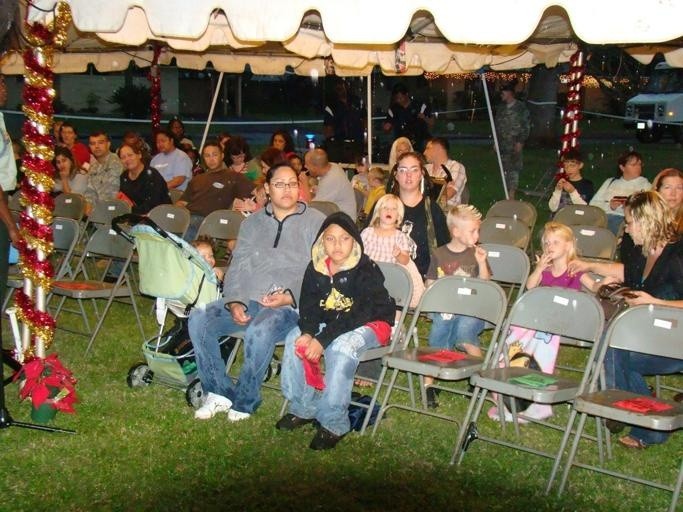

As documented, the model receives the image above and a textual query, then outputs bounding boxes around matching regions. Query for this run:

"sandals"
[618,434,649,449]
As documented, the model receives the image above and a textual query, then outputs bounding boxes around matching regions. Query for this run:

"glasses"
[271,181,300,188]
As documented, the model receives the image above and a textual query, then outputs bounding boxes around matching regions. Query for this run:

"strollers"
[109,208,280,410]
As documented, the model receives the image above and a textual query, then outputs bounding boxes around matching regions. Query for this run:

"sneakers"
[194,391,252,421]
[275,412,340,450]
[424,387,438,408]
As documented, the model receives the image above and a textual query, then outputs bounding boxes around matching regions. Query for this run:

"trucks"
[623,60,683,142]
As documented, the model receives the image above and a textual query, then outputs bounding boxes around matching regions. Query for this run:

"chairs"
[0,163,682,511]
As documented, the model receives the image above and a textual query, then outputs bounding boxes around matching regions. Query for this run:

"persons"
[323,117,352,161]
[486,221,620,421]
[274,211,396,450]
[352,137,468,340]
[188,165,328,421]
[13,119,203,283]
[174,129,356,243]
[548,149,683,235]
[189,241,223,281]
[425,204,492,408]
[323,78,364,153]
[385,82,434,153]
[568,191,682,451]
[1,73,20,357]
[490,86,530,199]
